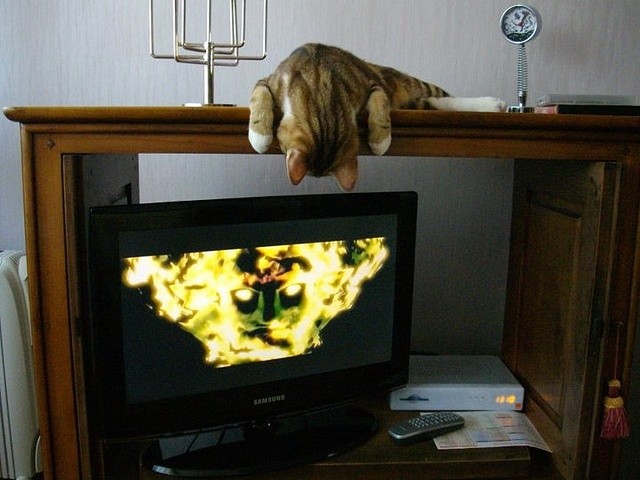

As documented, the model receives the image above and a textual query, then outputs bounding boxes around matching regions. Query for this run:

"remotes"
[386,412,470,440]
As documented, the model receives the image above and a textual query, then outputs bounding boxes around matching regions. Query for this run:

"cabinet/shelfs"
[2,106,640,480]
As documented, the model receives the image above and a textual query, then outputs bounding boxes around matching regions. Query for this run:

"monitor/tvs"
[84,190,415,480]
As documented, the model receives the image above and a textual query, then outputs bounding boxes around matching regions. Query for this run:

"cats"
[248,42,454,194]
